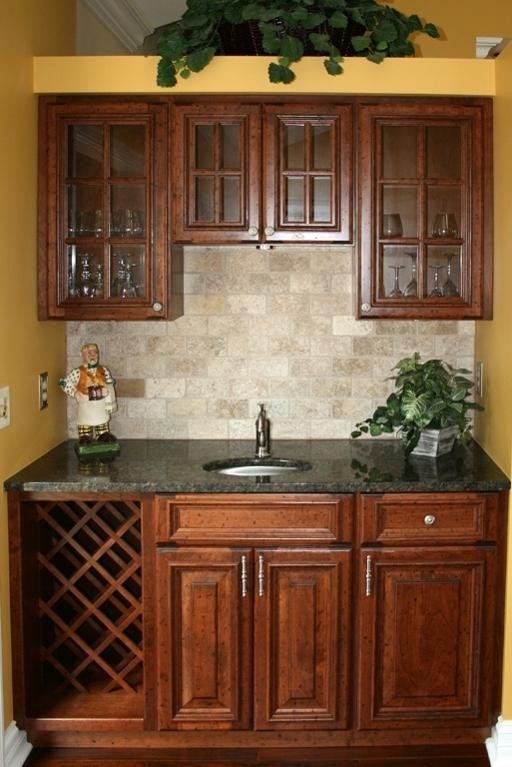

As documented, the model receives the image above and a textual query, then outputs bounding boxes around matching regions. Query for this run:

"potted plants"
[352,353,486,458]
[154,0,441,87]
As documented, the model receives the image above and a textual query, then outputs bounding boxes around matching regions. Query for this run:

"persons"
[59,344,118,444]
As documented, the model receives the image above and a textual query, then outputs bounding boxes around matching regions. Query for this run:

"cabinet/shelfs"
[170,100,355,245]
[358,105,487,321]
[43,97,168,318]
[150,494,352,732]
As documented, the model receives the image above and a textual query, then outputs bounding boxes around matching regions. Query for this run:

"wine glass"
[76,252,96,297]
[112,252,134,296]
[120,263,141,297]
[403,251,418,299]
[441,251,460,300]
[91,263,104,297]
[427,263,446,299]
[387,263,407,298]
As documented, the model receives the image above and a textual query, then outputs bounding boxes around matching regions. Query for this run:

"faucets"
[254,403,272,458]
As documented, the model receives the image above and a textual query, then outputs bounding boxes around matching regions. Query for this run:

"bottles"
[382,213,403,239]
[68,209,143,239]
[432,213,459,239]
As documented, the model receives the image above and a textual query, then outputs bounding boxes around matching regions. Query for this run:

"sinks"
[202,458,311,477]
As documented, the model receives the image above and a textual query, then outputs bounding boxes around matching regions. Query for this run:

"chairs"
[353,491,502,730]
